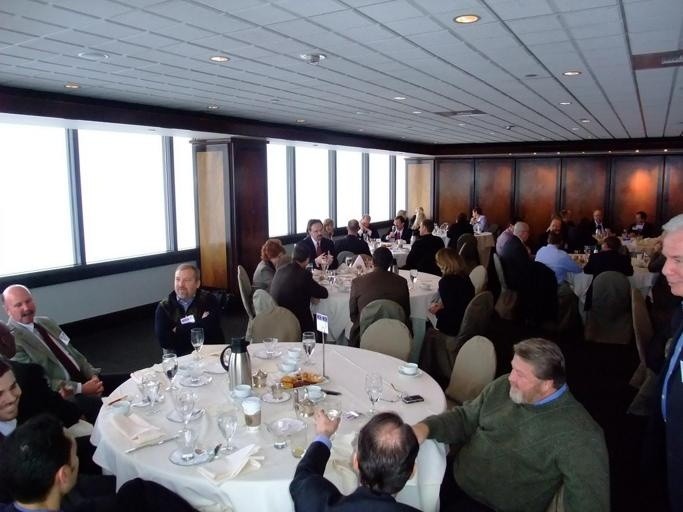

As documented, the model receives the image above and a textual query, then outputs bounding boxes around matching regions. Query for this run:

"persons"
[1,284,133,426]
[152,264,225,356]
[249,206,683,383]
[657,212,683,511]
[413,337,610,512]
[0,361,25,504]
[288,408,423,512]
[1,320,96,435]
[0,414,139,512]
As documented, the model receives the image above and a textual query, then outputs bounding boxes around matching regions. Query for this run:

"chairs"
[444,336,496,411]
[545,478,566,512]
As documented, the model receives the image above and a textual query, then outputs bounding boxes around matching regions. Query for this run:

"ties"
[316,241,322,257]
[397,230,400,239]
[32,321,82,383]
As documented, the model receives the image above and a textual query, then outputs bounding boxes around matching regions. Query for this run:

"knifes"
[209,444,222,462]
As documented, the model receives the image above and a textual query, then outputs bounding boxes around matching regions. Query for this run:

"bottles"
[623,229,627,237]
[292,388,309,408]
[410,231,416,244]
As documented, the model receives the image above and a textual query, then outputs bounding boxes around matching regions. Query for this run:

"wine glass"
[305,258,363,284]
[410,270,418,289]
[392,226,397,234]
[584,246,590,255]
[302,331,316,366]
[175,389,238,464]
[191,328,204,353]
[143,354,179,416]
[325,372,384,439]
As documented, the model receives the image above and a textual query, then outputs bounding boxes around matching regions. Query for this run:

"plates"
[311,391,327,403]
[262,391,291,403]
[266,417,306,434]
[166,407,205,423]
[397,369,423,377]
[179,359,226,387]
[169,446,208,466]
[252,343,280,358]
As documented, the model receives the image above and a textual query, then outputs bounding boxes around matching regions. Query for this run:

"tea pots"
[219,338,252,389]
[387,258,399,275]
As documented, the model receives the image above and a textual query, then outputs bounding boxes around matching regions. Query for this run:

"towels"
[106,413,165,446]
[100,395,128,405]
[331,431,359,496]
[198,443,265,487]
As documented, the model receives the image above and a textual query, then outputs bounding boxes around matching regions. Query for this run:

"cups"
[280,347,301,372]
[263,337,278,353]
[398,239,403,248]
[307,386,322,398]
[112,401,130,414]
[274,421,308,458]
[233,384,261,431]
[399,362,418,374]
[376,239,381,247]
[226,372,272,397]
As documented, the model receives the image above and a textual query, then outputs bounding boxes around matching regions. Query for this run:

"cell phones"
[402,394,424,404]
